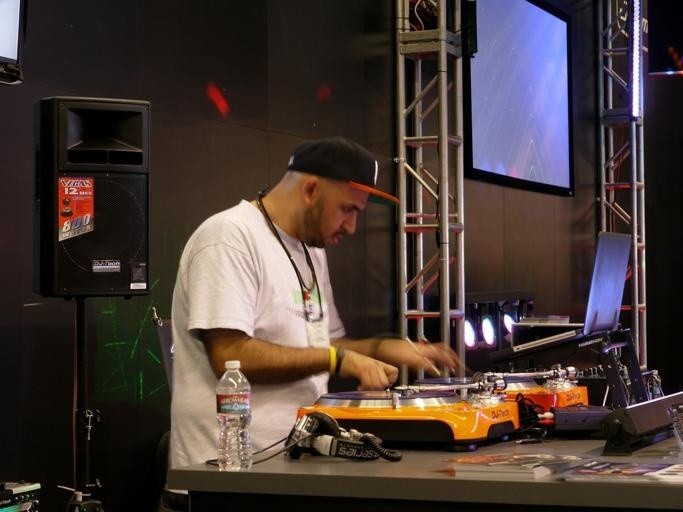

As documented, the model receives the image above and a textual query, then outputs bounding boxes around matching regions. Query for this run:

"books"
[443,451,682,483]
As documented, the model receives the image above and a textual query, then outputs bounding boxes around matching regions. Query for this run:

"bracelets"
[329,344,336,378]
[333,346,343,376]
[367,337,383,357]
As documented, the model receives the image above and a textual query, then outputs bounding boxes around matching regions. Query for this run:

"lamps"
[453,296,533,351]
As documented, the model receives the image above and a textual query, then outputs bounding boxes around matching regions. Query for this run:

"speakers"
[33,95,153,301]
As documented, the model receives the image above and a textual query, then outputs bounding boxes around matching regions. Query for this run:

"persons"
[160,136,463,511]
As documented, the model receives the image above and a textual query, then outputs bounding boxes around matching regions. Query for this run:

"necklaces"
[258,184,325,323]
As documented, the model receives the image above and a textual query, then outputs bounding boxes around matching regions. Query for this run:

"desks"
[165,436,683,511]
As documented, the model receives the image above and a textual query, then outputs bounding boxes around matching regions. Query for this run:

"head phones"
[287,411,384,460]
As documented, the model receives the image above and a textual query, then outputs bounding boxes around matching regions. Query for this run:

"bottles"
[215,360,251,471]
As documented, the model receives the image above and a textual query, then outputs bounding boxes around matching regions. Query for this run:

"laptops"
[488,231,632,360]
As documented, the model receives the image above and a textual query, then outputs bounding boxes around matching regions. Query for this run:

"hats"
[285,137,401,206]
